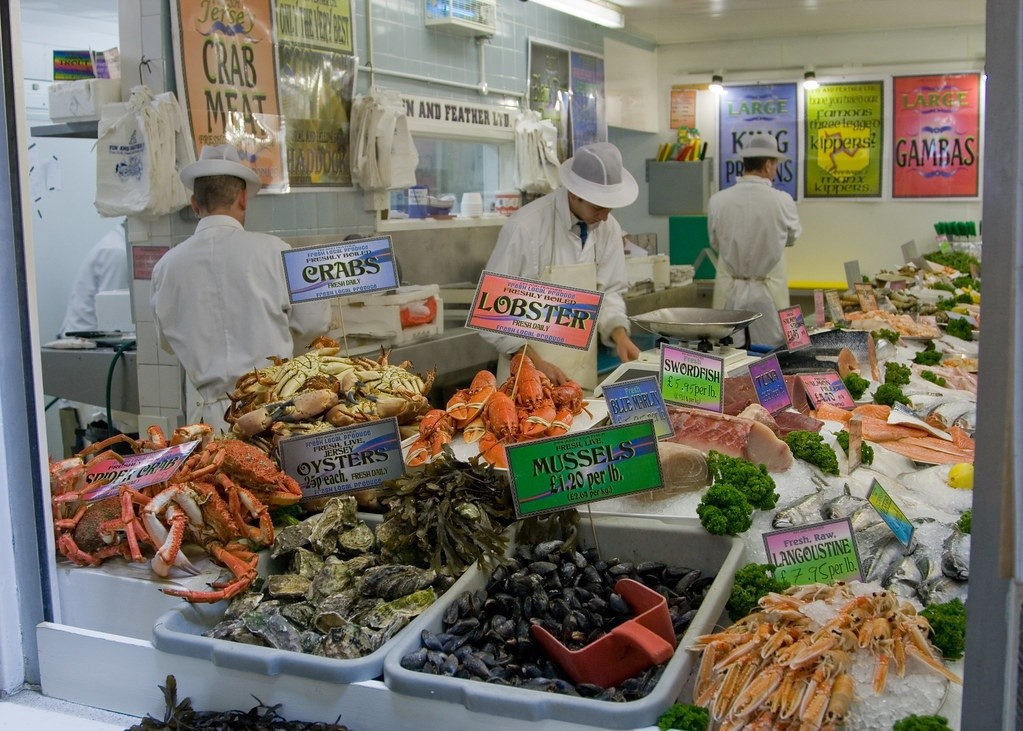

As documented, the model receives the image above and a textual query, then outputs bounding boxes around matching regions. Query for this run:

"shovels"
[529,576,680,689]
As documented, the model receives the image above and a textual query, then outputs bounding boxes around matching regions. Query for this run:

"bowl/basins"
[461,192,483,216]
[494,191,522,217]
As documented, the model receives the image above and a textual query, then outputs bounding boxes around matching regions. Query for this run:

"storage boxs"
[151,509,749,730]
[648,157,715,217]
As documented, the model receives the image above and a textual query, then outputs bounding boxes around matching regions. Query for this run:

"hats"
[179,143,260,199]
[557,141,639,208]
[738,133,785,164]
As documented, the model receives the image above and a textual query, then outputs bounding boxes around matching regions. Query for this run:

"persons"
[477,142,641,398]
[707,134,800,344]
[150,141,332,437]
[57,216,141,464]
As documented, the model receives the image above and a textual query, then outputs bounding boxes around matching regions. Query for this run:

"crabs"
[51,334,438,605]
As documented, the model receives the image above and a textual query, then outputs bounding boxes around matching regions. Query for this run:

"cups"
[651,253,670,291]
[408,185,428,218]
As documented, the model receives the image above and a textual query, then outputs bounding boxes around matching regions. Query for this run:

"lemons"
[949,463,973,490]
[972,293,981,303]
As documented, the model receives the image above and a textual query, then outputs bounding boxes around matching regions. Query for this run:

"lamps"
[530,0,625,30]
[707,69,726,95]
[801,64,820,91]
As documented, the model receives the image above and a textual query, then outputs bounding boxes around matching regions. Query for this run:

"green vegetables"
[656,248,983,731]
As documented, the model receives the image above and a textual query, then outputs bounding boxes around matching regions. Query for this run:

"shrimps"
[688,588,961,731]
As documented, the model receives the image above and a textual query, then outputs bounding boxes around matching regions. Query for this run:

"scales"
[593,306,764,399]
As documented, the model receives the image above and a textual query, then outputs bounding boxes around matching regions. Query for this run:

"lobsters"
[407,348,586,472]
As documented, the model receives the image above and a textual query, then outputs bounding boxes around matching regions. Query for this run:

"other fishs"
[772,472,971,593]
[807,379,975,472]
